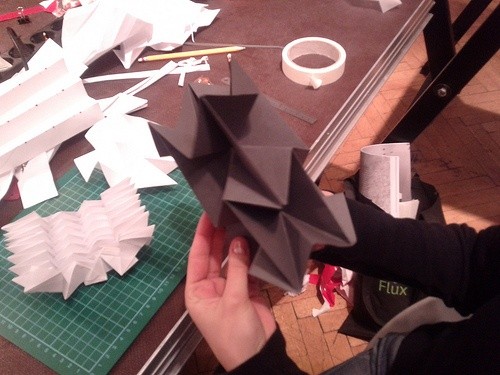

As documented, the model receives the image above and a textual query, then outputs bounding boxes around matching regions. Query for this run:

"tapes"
[280,36,347,88]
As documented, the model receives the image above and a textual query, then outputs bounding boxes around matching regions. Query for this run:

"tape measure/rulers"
[221,75,317,124]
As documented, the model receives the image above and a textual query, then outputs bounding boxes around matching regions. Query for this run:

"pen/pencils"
[138,46,246,62]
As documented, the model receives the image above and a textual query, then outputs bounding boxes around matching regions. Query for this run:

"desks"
[0,0,439,375]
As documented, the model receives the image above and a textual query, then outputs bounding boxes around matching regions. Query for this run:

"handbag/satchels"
[334,166,448,341]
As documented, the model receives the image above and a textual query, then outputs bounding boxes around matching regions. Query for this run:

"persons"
[182,186,500,374]
